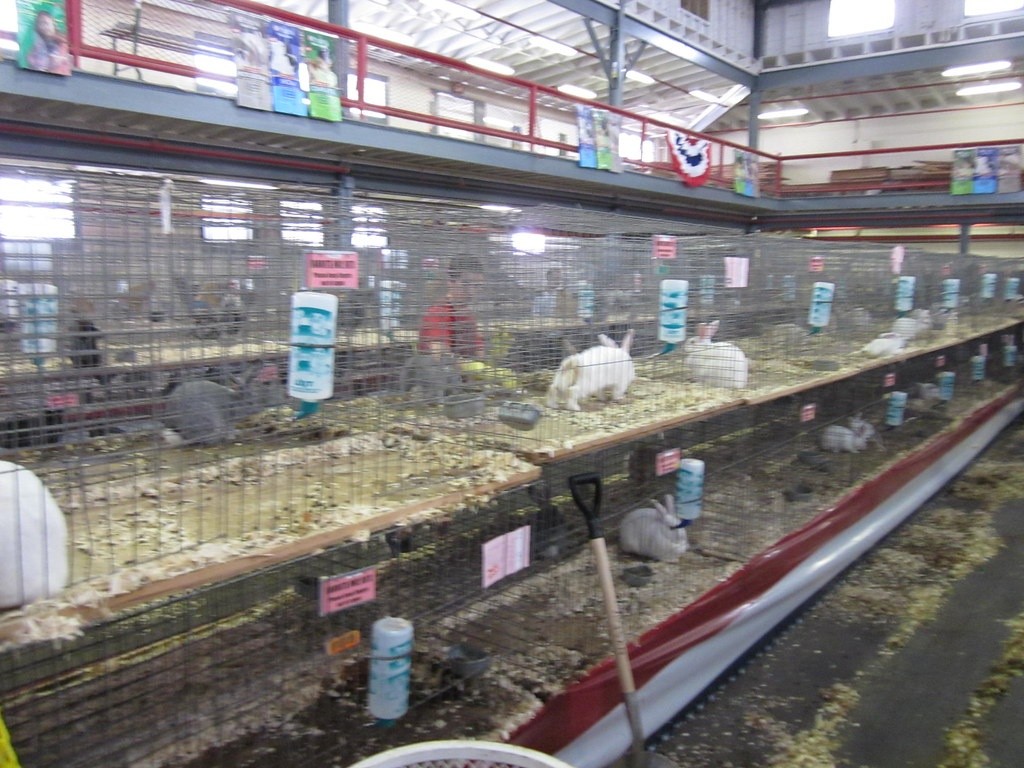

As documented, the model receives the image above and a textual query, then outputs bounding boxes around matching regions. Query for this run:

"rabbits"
[620,492,688,563]
[684,317,750,390]
[165,359,284,445]
[813,422,869,456]
[542,330,638,413]
[894,304,931,339]
[849,411,878,439]
[857,332,904,362]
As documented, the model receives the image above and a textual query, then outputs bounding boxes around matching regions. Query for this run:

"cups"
[370,618,413,719]
[809,282,835,327]
[699,275,716,303]
[887,392,907,426]
[782,275,796,301]
[380,281,403,315]
[1003,277,1020,300]
[971,356,986,379]
[937,372,956,401]
[896,276,915,311]
[289,290,337,400]
[20,285,60,352]
[943,279,960,307]
[577,283,596,318]
[675,458,706,519]
[980,274,997,298]
[659,280,688,343]
[1003,346,1018,366]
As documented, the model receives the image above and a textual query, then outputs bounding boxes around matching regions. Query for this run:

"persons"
[737,157,759,181]
[27,11,70,76]
[219,279,248,335]
[580,117,619,150]
[421,253,518,391]
[234,22,337,88]
[534,269,560,315]
[70,300,108,407]
[956,155,1024,179]
[342,282,366,329]
